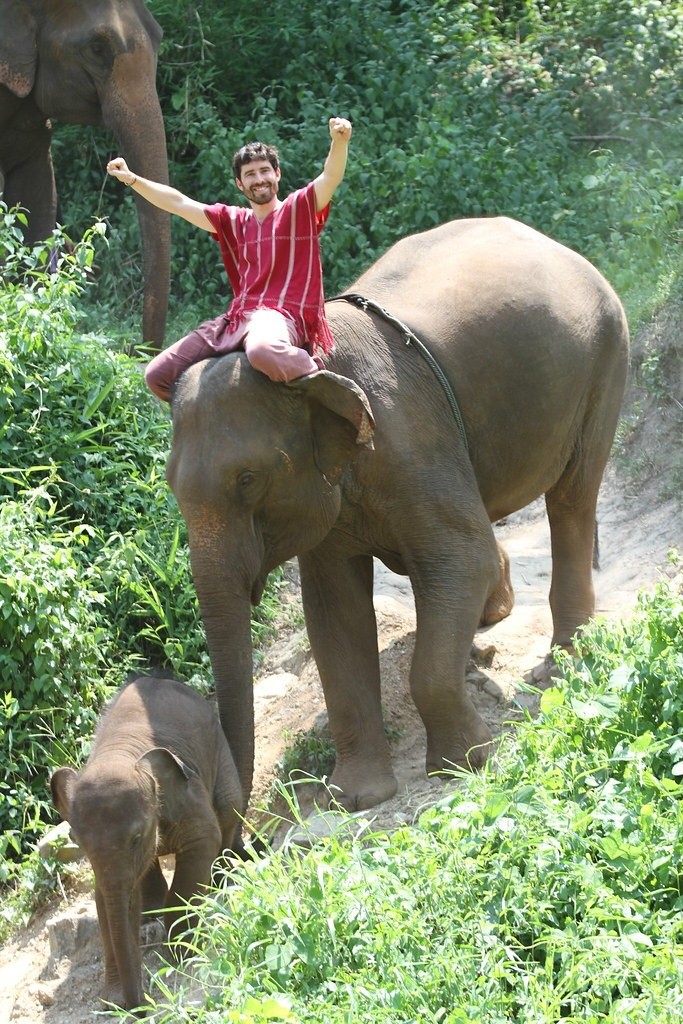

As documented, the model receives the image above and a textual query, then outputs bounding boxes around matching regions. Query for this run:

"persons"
[107,118,377,451]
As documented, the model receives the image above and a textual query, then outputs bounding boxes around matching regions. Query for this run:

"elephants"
[168,216,630,857]
[0,1,170,358]
[49,675,243,1017]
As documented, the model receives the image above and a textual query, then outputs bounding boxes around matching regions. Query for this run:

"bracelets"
[125,176,136,186]
